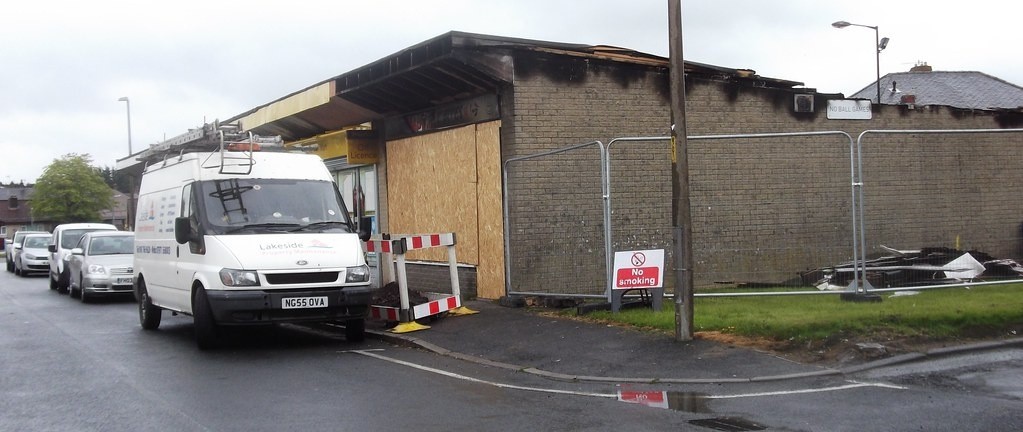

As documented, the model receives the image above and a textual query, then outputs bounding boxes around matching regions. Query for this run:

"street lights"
[118,96,133,157]
[832,21,891,104]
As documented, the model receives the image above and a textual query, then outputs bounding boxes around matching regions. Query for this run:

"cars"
[2,230,55,278]
[68,230,136,305]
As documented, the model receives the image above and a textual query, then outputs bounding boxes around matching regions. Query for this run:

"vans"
[130,119,372,353]
[47,223,123,295]
